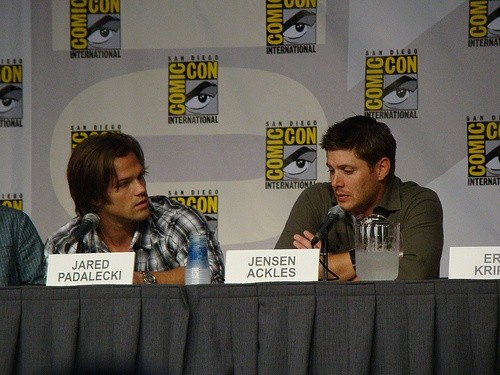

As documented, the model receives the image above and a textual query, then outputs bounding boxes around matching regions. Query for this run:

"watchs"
[140,270,157,285]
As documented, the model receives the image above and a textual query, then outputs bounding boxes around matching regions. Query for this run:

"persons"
[273,113,445,281]
[0,203,47,289]
[46,130,224,284]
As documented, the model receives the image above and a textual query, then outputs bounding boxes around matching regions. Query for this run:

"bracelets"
[348,249,356,272]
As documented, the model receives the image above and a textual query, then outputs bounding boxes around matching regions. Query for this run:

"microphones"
[311,205,346,246]
[65,213,101,253]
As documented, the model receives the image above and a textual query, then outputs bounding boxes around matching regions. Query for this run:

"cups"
[184,235,212,285]
[355,215,404,280]
